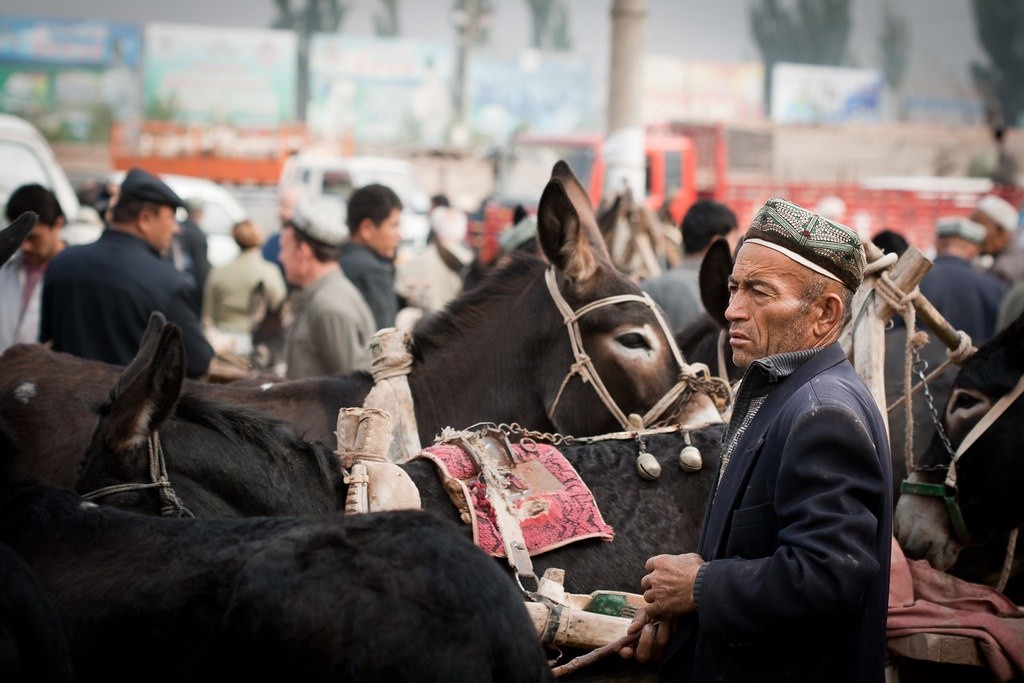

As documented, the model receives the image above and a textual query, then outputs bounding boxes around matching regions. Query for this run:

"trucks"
[461,114,994,286]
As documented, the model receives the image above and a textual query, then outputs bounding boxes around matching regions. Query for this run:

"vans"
[276,159,417,250]
[1,110,101,251]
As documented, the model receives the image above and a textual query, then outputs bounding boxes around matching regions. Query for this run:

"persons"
[642,200,739,331]
[920,215,1008,346]
[971,195,1024,285]
[201,220,287,358]
[336,183,399,332]
[620,197,893,683]
[0,183,70,356]
[425,195,450,244]
[427,206,472,296]
[279,200,376,379]
[255,193,297,345]
[176,197,208,280]
[39,168,214,381]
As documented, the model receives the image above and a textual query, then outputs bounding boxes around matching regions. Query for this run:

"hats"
[936,216,987,244]
[122,171,188,212]
[979,193,1019,232]
[744,194,868,294]
[292,201,349,248]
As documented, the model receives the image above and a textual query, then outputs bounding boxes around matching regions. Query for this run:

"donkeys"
[71,312,727,683]
[0,497,554,683]
[0,157,722,497]
[893,306,1024,606]
[671,227,1008,518]
